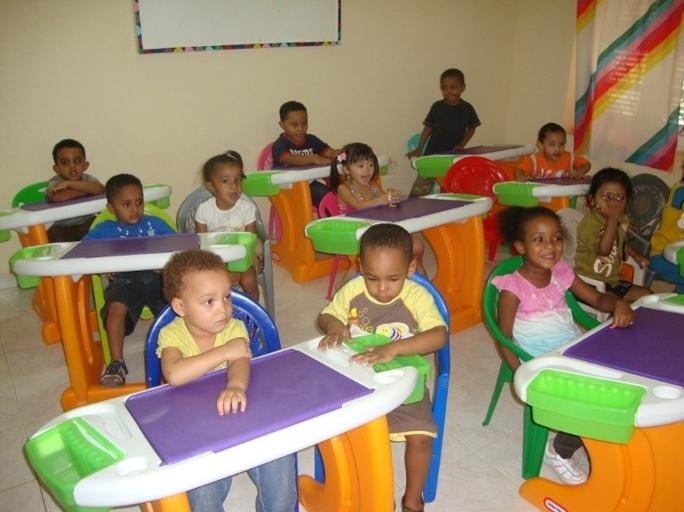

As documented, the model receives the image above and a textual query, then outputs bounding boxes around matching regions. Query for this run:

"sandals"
[100,361,128,385]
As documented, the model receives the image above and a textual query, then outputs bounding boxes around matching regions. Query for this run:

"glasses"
[601,192,624,202]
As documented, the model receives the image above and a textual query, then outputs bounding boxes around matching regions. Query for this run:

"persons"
[495,204,635,486]
[41,140,106,244]
[328,139,426,279]
[74,173,176,388]
[407,67,481,198]
[514,122,593,181]
[575,167,659,302]
[311,222,449,511]
[152,248,301,512]
[193,150,266,308]
[269,101,340,207]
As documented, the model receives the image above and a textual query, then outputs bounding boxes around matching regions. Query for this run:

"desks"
[512,290,683,512]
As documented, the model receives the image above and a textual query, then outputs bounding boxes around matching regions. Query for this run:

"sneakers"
[543,437,588,484]
